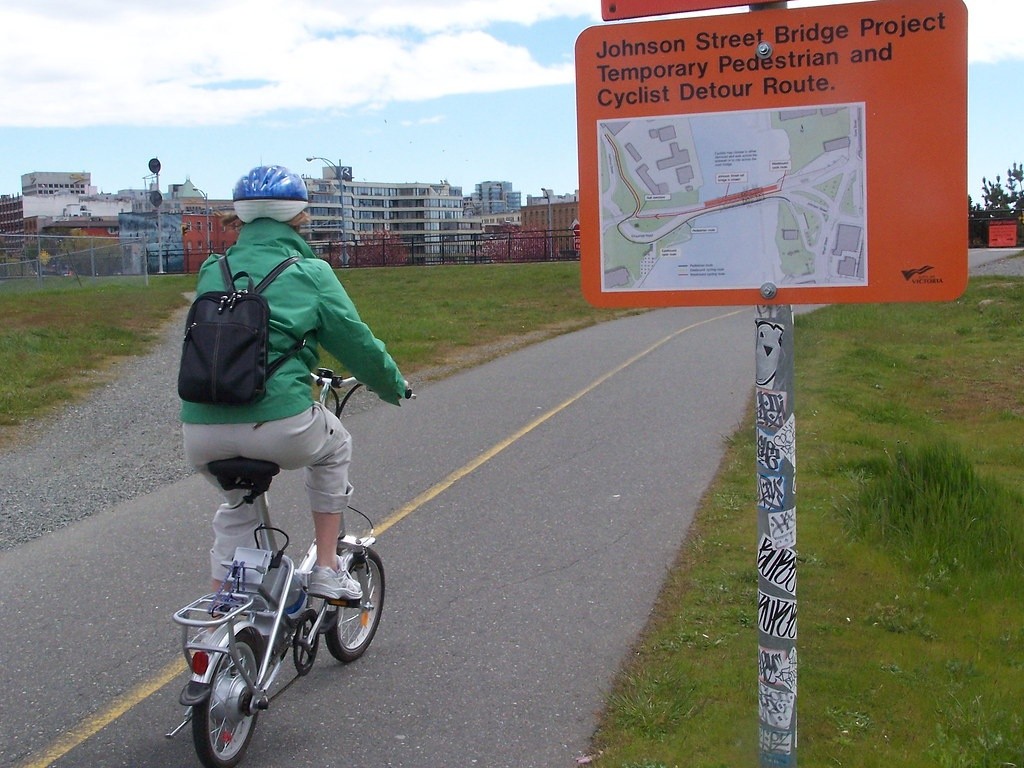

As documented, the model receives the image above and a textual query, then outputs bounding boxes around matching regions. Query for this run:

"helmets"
[233,165,311,224]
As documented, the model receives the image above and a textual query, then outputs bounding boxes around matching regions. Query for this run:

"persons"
[178,166,409,647]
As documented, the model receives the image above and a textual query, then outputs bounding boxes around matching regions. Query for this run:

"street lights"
[305,156,349,268]
[192,187,211,258]
[541,186,555,258]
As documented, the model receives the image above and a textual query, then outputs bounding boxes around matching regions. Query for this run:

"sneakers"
[306,554,363,600]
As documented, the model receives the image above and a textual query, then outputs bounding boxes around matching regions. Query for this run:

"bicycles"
[159,370,390,766]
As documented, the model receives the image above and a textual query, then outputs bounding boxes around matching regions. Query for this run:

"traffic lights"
[182,225,188,236]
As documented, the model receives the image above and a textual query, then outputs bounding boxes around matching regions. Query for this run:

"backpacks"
[178,254,311,407]
